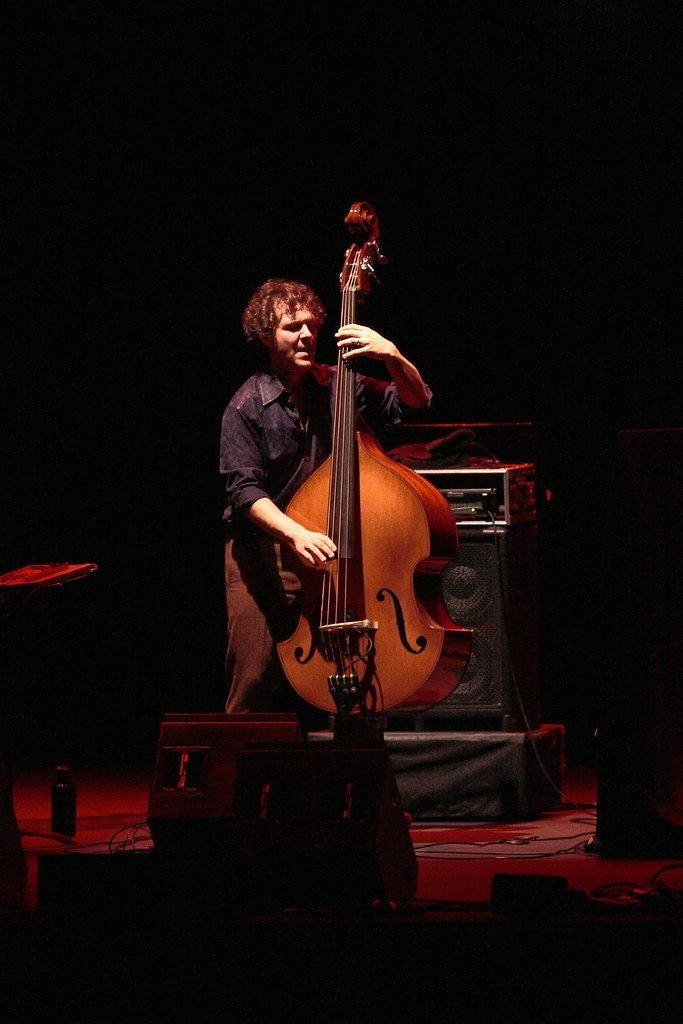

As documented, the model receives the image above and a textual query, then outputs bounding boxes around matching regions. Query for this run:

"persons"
[219,278,436,722]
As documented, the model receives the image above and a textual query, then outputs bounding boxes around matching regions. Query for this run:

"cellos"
[272,198,480,724]
